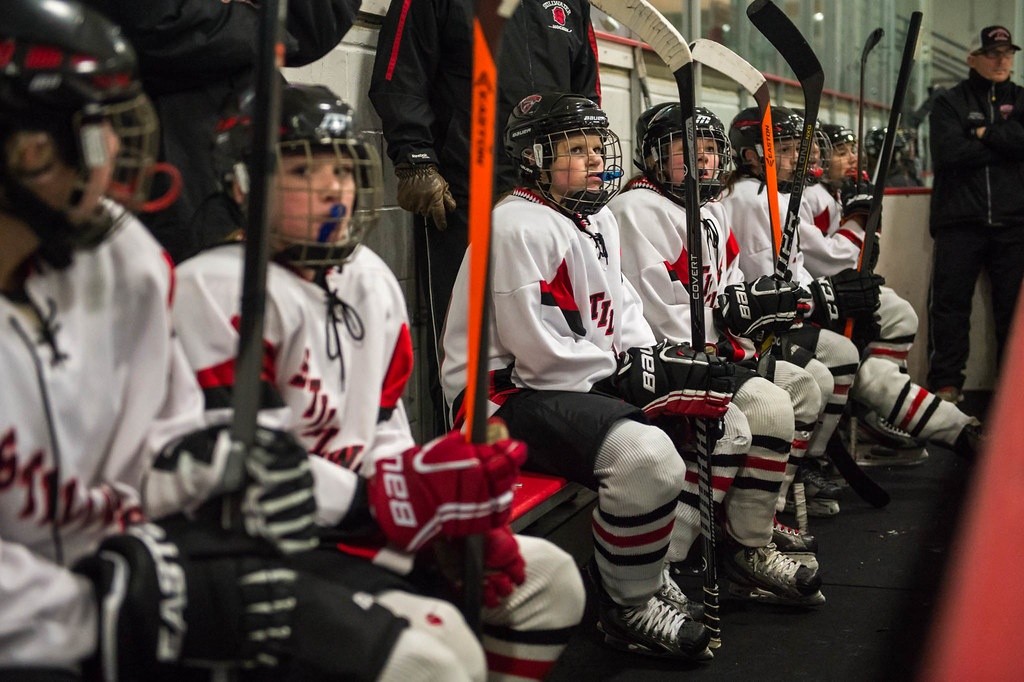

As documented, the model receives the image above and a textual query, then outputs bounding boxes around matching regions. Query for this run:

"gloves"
[838,176,883,218]
[366,414,526,555]
[395,164,456,232]
[404,526,526,608]
[809,266,884,352]
[614,337,734,460]
[72,504,298,682]
[731,278,810,355]
[142,422,321,556]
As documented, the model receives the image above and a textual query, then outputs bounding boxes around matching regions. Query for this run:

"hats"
[971,25,1022,56]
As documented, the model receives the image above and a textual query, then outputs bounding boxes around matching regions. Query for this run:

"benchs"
[505,475,588,536]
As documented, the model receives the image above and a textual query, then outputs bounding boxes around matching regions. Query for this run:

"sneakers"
[661,564,721,648]
[722,533,826,607]
[933,386,965,406]
[596,585,714,662]
[850,406,929,467]
[783,451,846,518]
[768,517,819,571]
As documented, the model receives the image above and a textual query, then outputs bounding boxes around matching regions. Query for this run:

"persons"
[926,26,1024,400]
[715,108,989,516]
[864,125,924,188]
[369,0,600,435]
[90,0,363,262]
[168,84,586,682]
[438,91,738,656]
[608,102,826,607]
[0,0,467,682]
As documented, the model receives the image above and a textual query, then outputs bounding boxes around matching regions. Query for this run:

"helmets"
[791,108,833,183]
[504,91,625,218]
[212,84,383,270]
[0,1,161,253]
[636,101,731,207]
[818,123,857,185]
[731,106,811,193]
[866,127,920,171]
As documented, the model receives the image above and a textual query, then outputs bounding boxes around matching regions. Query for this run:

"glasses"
[985,48,1016,60]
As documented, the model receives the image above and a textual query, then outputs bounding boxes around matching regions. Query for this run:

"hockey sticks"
[684,34,813,535]
[216,0,291,536]
[744,0,827,364]
[451,0,525,653]
[580,0,726,655]
[823,8,924,514]
[855,26,886,195]
